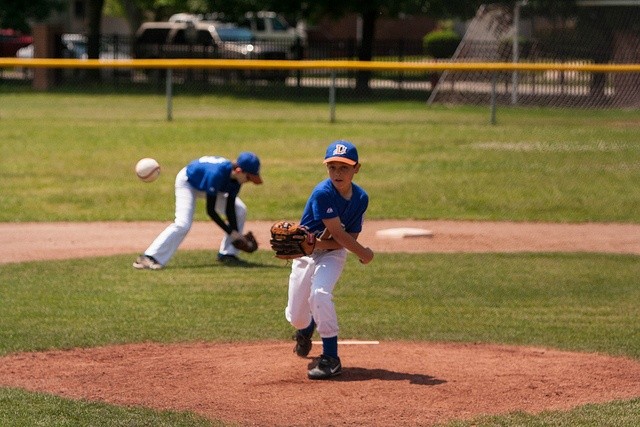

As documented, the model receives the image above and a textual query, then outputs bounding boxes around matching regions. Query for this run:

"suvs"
[134,20,293,84]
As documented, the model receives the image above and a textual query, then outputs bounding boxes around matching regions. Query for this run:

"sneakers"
[217,253,244,264]
[307,353,341,378]
[132,253,161,269]
[292,319,317,357]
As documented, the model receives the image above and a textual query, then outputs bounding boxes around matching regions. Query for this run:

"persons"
[281,141,373,379]
[130,151,263,270]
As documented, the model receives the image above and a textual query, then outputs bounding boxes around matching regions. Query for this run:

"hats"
[323,140,358,165]
[237,151,263,184]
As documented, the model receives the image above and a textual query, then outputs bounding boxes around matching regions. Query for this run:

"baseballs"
[135,158,160,183]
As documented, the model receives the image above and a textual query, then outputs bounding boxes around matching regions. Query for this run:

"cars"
[16,34,135,81]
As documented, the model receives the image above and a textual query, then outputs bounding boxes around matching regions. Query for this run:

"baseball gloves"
[232,232,258,253]
[270,221,316,259]
